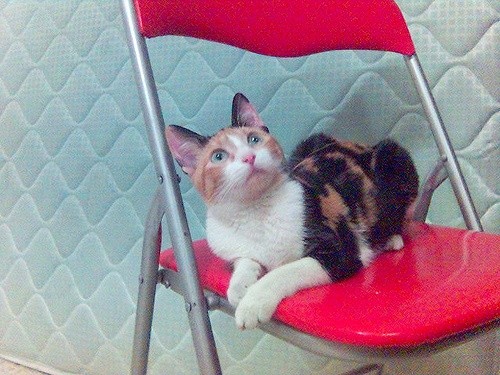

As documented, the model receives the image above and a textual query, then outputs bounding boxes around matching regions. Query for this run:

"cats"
[164,93,419,330]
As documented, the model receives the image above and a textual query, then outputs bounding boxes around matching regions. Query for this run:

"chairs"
[116,0,499,375]
